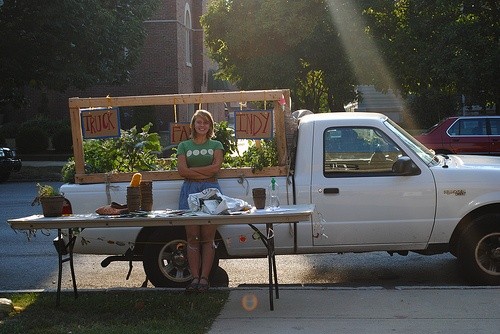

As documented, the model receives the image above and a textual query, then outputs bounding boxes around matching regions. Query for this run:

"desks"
[7,204,315,311]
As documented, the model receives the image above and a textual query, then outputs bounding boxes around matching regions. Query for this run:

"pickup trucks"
[56,110,500,289]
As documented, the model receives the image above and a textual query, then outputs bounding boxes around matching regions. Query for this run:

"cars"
[0,144,23,184]
[410,114,500,157]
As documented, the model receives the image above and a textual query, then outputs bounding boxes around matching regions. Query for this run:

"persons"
[176,110,225,291]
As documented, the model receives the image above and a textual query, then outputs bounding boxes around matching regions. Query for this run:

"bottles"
[268,178,280,210]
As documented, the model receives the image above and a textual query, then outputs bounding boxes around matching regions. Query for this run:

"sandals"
[186,276,200,291]
[197,277,209,291]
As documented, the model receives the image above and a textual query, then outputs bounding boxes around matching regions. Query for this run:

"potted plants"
[31,183,65,216]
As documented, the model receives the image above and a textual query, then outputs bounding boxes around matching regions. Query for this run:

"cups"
[127,180,153,213]
[252,188,266,209]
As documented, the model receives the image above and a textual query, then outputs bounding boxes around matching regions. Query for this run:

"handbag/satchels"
[95,201,128,215]
[188,188,252,215]
